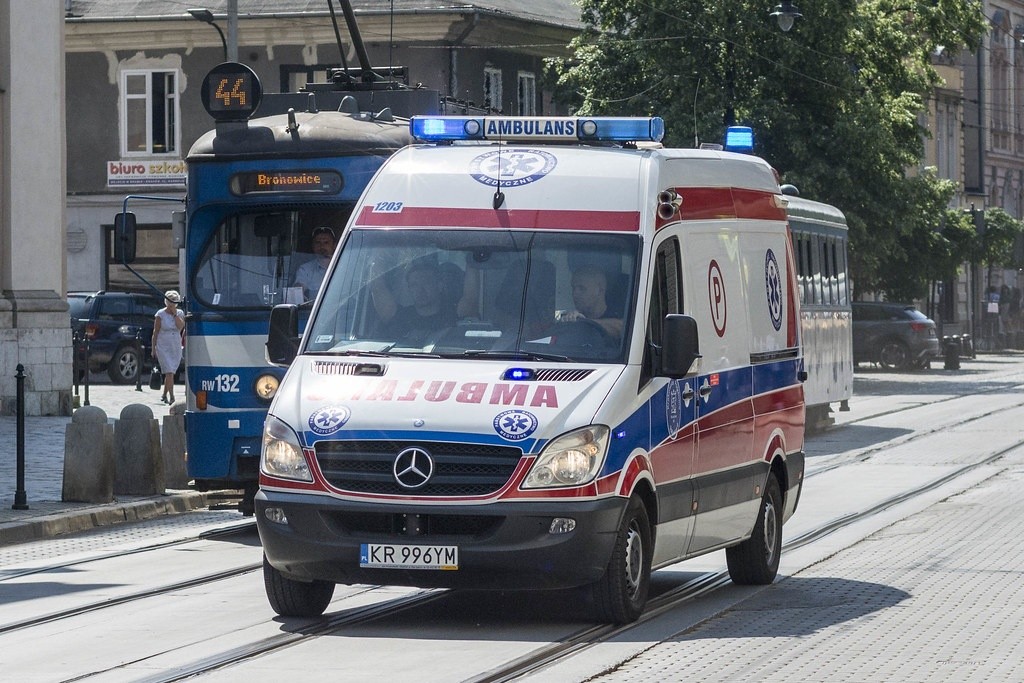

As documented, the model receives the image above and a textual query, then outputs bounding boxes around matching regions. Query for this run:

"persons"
[151,290,185,404]
[561,263,626,348]
[369,263,483,349]
[293,226,338,305]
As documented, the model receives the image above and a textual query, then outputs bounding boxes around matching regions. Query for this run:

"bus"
[114,0,853,516]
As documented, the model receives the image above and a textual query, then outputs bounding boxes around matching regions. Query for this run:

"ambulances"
[254,115,808,616]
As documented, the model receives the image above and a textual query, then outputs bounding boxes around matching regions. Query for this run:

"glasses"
[311,224,336,237]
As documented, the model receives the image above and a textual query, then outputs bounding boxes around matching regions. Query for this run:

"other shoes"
[161,395,176,405]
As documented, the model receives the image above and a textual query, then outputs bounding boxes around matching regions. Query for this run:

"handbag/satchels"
[149,355,162,390]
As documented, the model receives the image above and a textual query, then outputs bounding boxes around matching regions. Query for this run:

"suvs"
[851,301,939,370]
[67,290,185,385]
